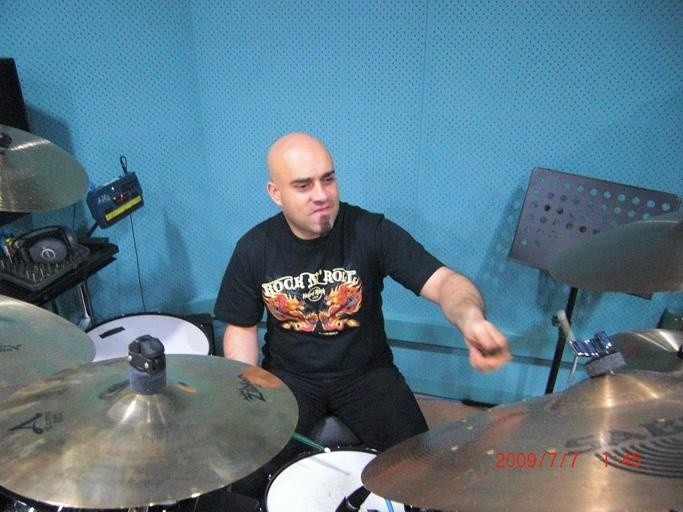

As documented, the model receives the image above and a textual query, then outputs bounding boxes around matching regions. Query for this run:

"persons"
[214,134,510,496]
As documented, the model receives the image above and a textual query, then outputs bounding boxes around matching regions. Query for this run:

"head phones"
[11,225,78,265]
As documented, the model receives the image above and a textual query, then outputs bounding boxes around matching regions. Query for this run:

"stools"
[291,415,362,454]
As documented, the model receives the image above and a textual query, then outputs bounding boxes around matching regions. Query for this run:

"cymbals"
[1,119,90,213]
[1,290,96,390]
[361,366,682,511]
[0,354,299,512]
[548,208,683,294]
[606,329,683,373]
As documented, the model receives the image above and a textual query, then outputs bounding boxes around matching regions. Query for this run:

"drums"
[86,312,212,362]
[262,442,413,512]
